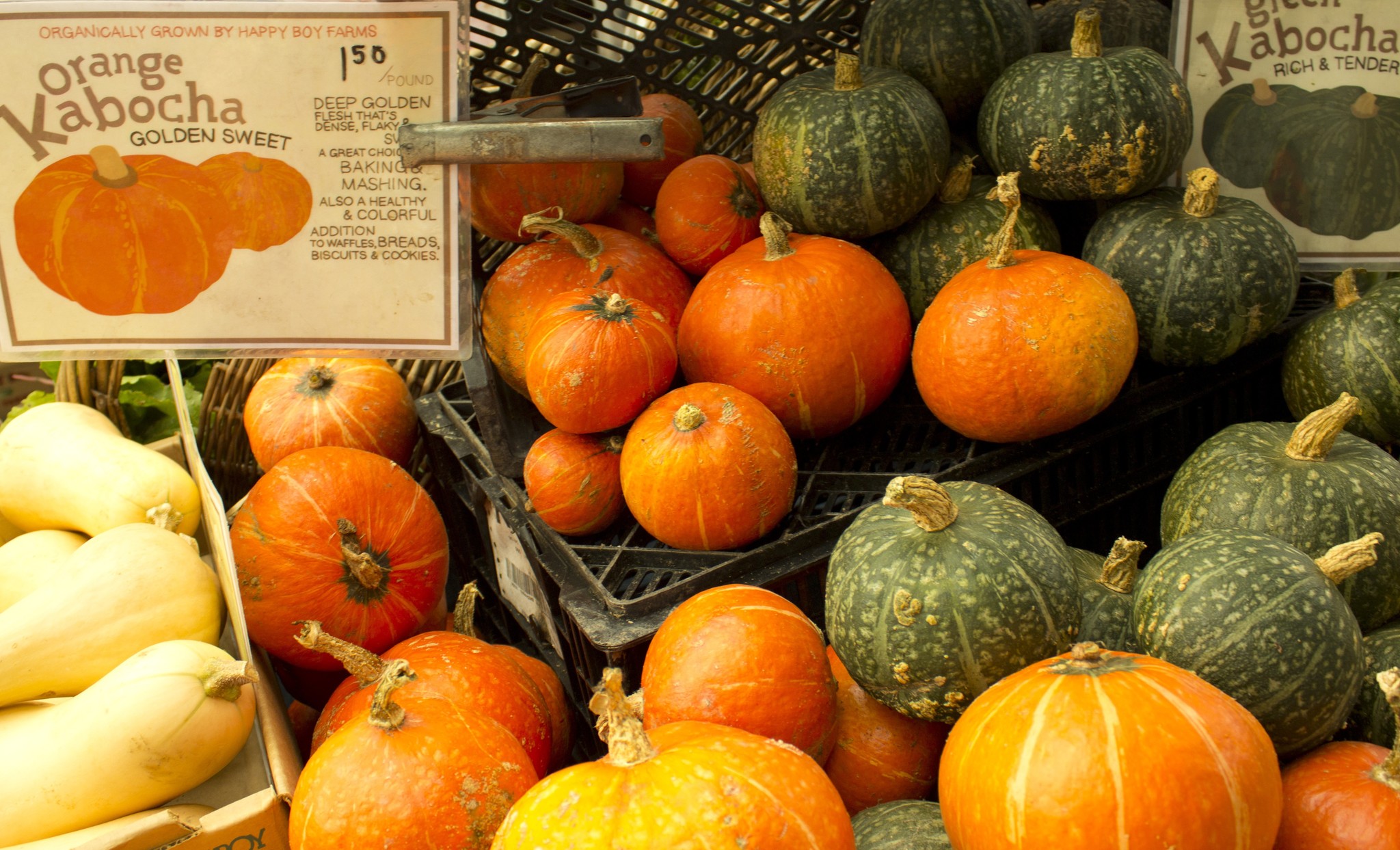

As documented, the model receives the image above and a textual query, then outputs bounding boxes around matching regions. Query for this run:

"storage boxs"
[445,0,882,481]
[0,436,308,850]
[406,265,1349,768]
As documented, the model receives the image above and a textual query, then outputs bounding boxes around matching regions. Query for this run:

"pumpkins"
[1,1,1397,849]
